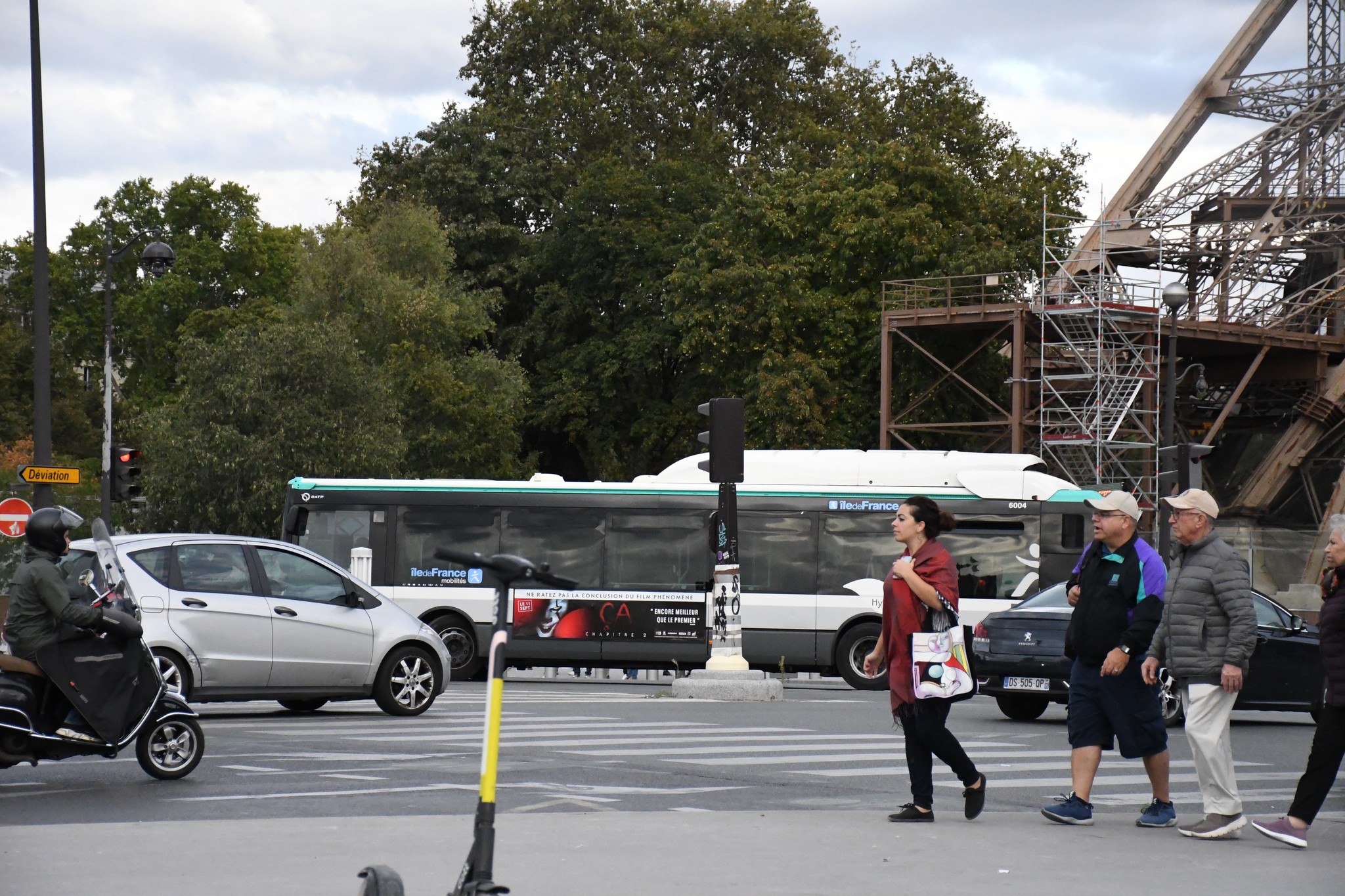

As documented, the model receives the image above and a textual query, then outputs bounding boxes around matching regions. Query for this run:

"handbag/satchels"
[907,604,978,705]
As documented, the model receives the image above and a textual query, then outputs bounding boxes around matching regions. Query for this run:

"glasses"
[1092,511,1125,520]
[1170,511,1206,520]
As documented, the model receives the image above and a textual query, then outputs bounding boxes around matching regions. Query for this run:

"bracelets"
[1117,644,1132,656]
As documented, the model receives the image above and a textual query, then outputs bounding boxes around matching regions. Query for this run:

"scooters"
[0,517,205,781]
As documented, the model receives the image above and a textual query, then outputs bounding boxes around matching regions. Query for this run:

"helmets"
[25,508,73,554]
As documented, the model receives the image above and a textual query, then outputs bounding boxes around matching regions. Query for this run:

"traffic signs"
[16,463,81,484]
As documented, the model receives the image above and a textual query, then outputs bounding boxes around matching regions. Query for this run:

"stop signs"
[0,497,33,539]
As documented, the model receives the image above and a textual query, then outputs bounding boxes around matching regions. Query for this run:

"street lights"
[1159,281,1208,575]
[98,228,179,538]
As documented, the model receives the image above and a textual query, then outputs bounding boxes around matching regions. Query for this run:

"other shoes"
[557,669,559,674]
[585,673,590,678]
[606,675,609,679]
[572,674,578,678]
[628,677,637,680]
[663,670,672,676]
[624,676,629,679]
[568,671,575,675]
[56,723,104,742]
[622,674,627,679]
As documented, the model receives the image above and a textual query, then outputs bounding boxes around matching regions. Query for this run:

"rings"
[1114,669,1118,672]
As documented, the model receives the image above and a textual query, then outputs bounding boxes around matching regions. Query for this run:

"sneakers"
[1251,815,1308,848]
[889,803,934,823]
[1040,791,1094,826]
[962,772,986,820]
[1178,814,1241,839]
[1136,796,1178,827]
[1191,812,1247,837]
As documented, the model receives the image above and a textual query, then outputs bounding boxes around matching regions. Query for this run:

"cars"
[971,568,1329,726]
[59,532,450,718]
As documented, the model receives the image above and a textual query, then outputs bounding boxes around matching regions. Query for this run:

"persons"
[1042,490,1180,827]
[864,495,986,822]
[205,553,246,593]
[1141,488,1258,840]
[2,507,143,742]
[1251,515,1345,850]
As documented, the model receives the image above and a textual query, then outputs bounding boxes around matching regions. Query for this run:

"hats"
[1162,488,1219,519]
[1083,490,1140,522]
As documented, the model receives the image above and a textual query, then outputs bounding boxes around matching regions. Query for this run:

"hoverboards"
[359,546,581,896]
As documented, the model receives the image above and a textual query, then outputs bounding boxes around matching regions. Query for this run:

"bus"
[284,448,1114,692]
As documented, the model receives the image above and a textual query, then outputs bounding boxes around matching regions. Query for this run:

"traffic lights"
[1157,445,1178,499]
[697,398,744,484]
[112,443,143,503]
[1177,443,1216,491]
